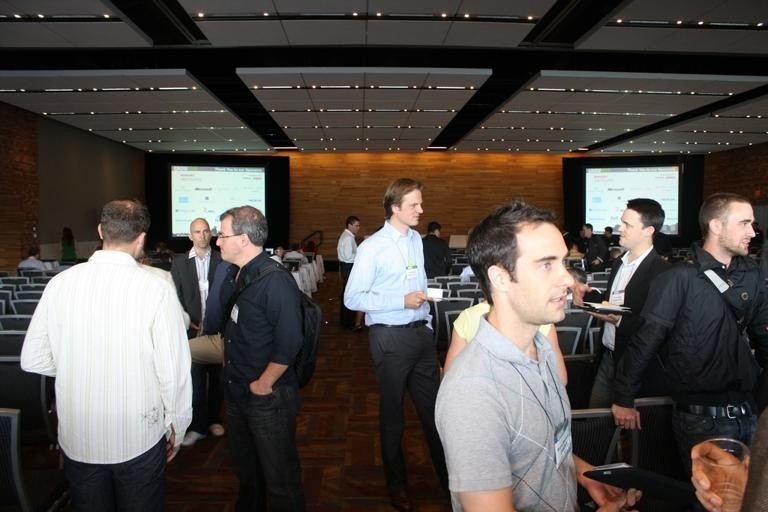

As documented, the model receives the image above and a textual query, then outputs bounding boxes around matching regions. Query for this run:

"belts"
[603,346,614,359]
[673,401,757,418]
[369,320,428,328]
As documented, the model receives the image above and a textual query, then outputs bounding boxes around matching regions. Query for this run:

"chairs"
[31,276,53,283]
[43,271,60,276]
[287,258,302,268]
[570,409,625,468]
[428,289,452,329]
[0,408,68,512]
[0,285,16,289]
[444,311,465,346]
[670,246,695,264]
[455,257,468,264]
[451,254,465,259]
[567,258,582,268]
[555,309,592,354]
[0,330,27,356]
[556,327,582,355]
[605,268,613,273]
[0,290,13,298]
[478,298,486,303]
[434,276,462,282]
[447,282,479,290]
[589,327,607,373]
[427,283,442,288]
[282,261,299,271]
[14,290,43,299]
[1,314,34,330]
[0,300,5,313]
[19,284,45,289]
[632,397,695,496]
[434,298,474,349]
[563,355,596,409]
[467,274,479,283]
[1,276,29,283]
[451,264,469,275]
[587,281,608,287]
[1,356,58,446]
[9,299,40,314]
[19,270,43,276]
[591,272,611,281]
[457,289,485,306]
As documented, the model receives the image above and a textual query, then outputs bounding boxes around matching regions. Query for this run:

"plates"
[428,298,448,302]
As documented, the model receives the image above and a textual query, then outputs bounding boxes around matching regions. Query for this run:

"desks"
[312,260,320,285]
[304,262,318,293]
[292,271,305,292]
[316,257,323,283]
[298,268,312,297]
[318,254,324,274]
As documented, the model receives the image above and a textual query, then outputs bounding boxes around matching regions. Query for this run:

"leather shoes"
[390,483,412,512]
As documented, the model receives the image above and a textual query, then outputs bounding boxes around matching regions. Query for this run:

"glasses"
[216,232,243,238]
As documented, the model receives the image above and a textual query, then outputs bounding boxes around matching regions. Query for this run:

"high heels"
[353,325,362,332]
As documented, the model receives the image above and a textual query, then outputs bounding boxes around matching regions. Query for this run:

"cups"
[698,438,751,512]
[427,288,443,302]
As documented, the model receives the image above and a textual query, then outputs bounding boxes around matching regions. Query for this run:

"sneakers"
[182,432,206,445]
[210,423,224,436]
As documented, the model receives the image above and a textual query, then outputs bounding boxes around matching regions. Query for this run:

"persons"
[217,205,308,512]
[603,227,617,247]
[560,226,572,251]
[155,241,176,266]
[578,228,587,254]
[583,224,606,272]
[18,247,46,276]
[344,178,451,512]
[20,199,192,512]
[422,222,452,278]
[750,221,763,246]
[284,242,307,264]
[691,409,767,512]
[59,226,79,262]
[612,193,768,486]
[336,217,362,336]
[171,218,226,447]
[435,202,641,512]
[303,240,319,261]
[269,246,284,265]
[442,301,568,388]
[188,261,231,366]
[588,199,671,413]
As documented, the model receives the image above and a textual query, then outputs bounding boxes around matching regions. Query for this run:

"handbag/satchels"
[702,274,768,418]
[218,264,322,388]
